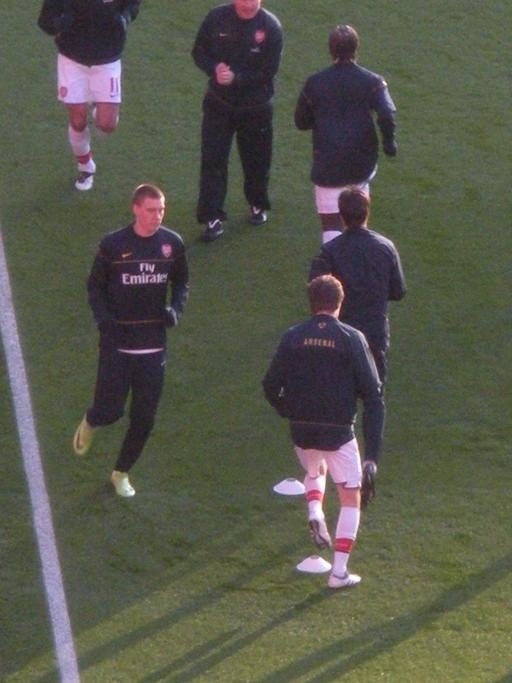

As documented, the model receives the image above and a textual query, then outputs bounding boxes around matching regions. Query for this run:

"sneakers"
[202,218,223,241]
[328,573,363,589]
[361,461,376,507]
[309,510,331,550]
[73,418,96,455]
[75,171,94,190]
[250,204,268,225]
[109,471,135,498]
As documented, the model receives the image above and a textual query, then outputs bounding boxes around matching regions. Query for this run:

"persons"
[72,183,191,499]
[37,0,144,191]
[191,0,287,242]
[294,23,399,244]
[262,271,388,590]
[307,183,409,508]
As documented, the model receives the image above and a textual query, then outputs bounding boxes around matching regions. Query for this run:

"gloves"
[384,141,398,157]
